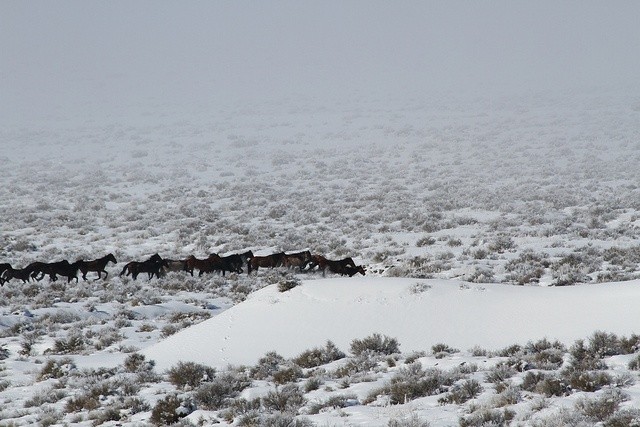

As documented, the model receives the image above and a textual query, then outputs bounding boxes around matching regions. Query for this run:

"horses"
[131,258,169,283]
[77,252,117,282]
[165,255,196,280]
[249,251,289,278]
[32,259,67,285]
[216,253,244,280]
[340,264,366,278]
[118,252,163,277]
[191,252,221,279]
[0,262,12,287]
[236,249,254,265]
[306,255,331,278]
[286,250,311,274]
[56,260,84,286]
[2,262,40,289]
[328,257,355,277]
